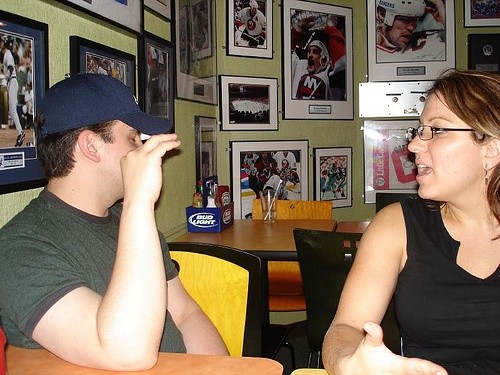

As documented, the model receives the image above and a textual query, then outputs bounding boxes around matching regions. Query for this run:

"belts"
[7,76,17,84]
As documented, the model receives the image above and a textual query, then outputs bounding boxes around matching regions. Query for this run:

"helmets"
[376,0,426,26]
[249,0,258,10]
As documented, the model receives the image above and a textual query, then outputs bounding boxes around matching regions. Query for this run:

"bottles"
[197,181,204,197]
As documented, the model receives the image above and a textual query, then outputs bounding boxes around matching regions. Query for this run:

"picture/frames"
[0,0,500,221]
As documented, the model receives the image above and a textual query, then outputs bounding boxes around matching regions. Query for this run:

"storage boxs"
[186,202,233,232]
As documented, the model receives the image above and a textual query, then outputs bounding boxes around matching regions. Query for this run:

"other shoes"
[332,192,336,197]
[320,192,323,199]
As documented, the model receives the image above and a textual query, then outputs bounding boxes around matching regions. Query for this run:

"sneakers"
[15,130,26,147]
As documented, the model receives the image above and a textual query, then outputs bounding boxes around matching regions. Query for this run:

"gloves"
[258,32,265,44]
[234,19,242,27]
[0,79,7,87]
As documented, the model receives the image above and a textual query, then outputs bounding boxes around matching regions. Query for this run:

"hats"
[39,73,173,135]
[307,28,331,59]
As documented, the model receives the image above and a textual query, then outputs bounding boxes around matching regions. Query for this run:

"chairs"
[292,226,398,369]
[166,241,262,356]
[248,198,334,312]
[168,249,248,357]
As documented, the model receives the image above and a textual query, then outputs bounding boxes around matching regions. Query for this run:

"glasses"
[405,123,478,141]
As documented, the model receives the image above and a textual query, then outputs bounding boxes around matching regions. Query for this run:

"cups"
[259,189,277,223]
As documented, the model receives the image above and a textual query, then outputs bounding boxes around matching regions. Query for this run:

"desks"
[0,218,371,375]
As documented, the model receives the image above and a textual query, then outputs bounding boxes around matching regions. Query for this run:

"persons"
[321,68,500,375]
[0,72,231,371]
[0,0,446,200]
